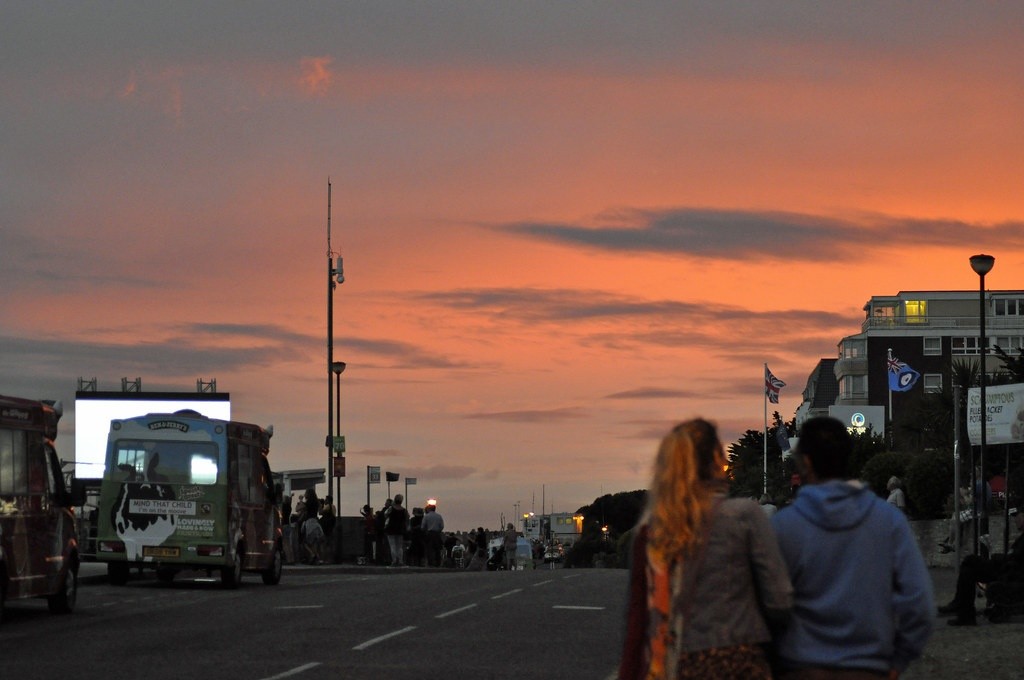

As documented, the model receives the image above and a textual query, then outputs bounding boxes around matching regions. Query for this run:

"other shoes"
[937,602,977,626]
[310,553,321,564]
[391,562,406,567]
[938,538,955,554]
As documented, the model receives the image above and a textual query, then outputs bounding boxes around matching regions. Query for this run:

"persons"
[444,533,465,569]
[886,476,905,512]
[502,523,518,570]
[281,489,336,565]
[617,418,794,680]
[360,494,444,567]
[937,512,1024,625]
[938,465,992,554]
[467,527,487,570]
[769,416,936,680]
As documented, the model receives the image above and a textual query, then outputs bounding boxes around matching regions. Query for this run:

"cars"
[544,549,562,564]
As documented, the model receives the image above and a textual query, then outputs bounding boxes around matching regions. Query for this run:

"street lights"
[969,254,995,558]
[523,513,529,535]
[330,361,347,541]
[602,526,608,543]
[572,514,584,540]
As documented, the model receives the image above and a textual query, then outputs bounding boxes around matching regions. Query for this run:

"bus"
[95,408,285,587]
[0,395,89,617]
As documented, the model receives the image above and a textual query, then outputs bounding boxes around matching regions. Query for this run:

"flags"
[886,356,920,392]
[765,367,786,404]
